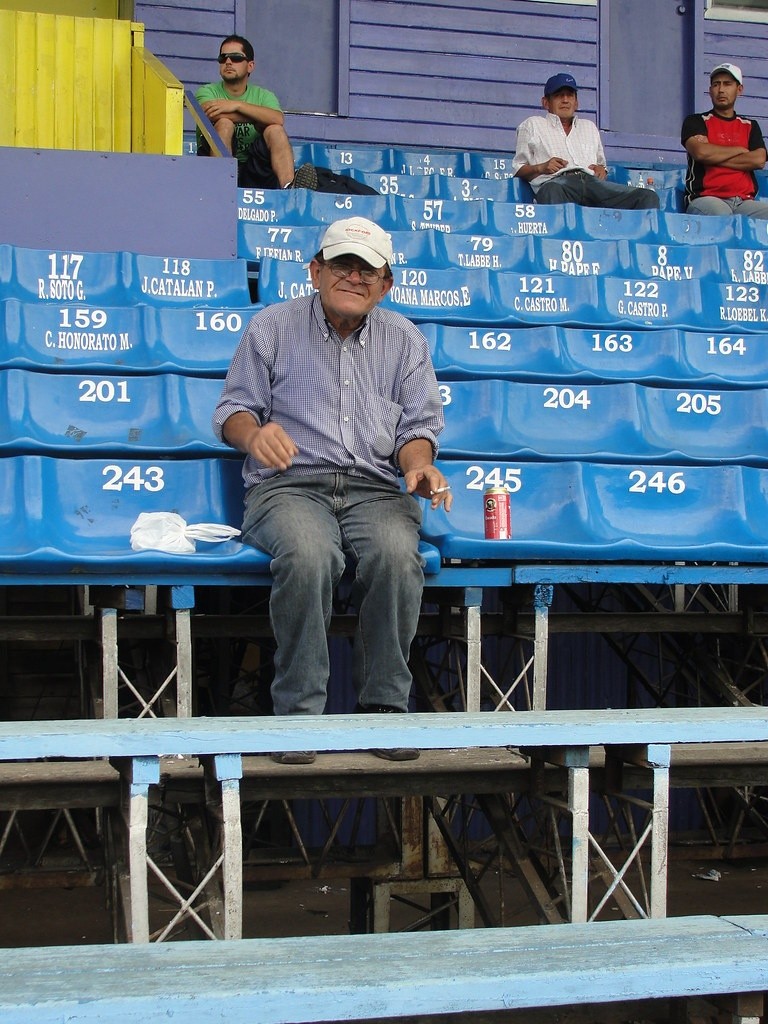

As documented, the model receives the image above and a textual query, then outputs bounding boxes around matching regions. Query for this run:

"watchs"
[599,165,609,174]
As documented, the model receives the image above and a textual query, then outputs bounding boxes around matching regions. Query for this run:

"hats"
[710,63,743,85]
[318,216,393,268]
[544,73,578,96]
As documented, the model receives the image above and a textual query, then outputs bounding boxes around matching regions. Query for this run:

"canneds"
[483,487,511,540]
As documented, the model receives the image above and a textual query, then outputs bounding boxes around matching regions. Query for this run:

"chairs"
[0,130,768,720]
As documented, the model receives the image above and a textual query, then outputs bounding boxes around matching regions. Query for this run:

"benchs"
[0,703,768,1024]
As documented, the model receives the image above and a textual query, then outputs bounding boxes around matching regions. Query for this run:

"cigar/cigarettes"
[431,485,451,496]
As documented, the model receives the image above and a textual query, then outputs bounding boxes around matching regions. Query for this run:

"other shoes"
[284,163,318,191]
[357,705,421,761]
[270,750,314,763]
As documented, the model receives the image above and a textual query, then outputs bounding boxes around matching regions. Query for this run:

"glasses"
[218,53,250,64]
[320,262,387,284]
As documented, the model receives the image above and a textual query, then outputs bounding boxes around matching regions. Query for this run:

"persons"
[511,73,660,212]
[210,215,453,765]
[681,61,768,220]
[194,34,318,190]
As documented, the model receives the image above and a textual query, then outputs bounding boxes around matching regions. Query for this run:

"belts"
[561,169,584,175]
